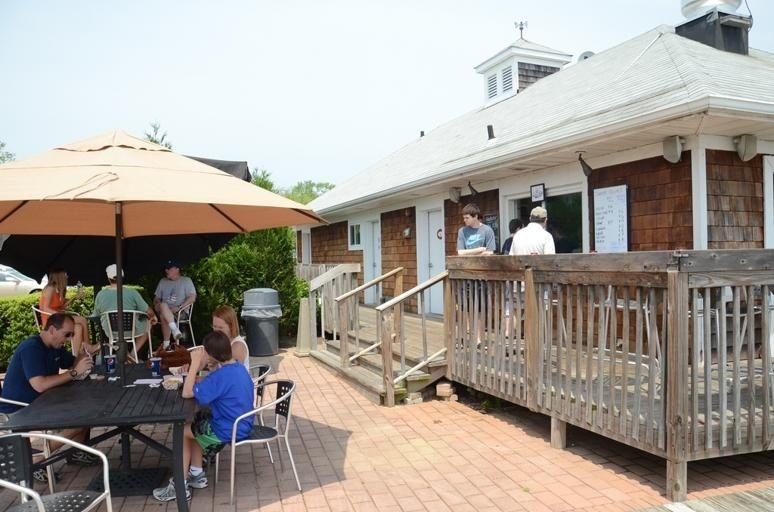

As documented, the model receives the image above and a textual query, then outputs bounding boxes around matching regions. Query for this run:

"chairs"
[215,378,303,505]
[31,303,81,357]
[0,376,65,484]
[0,432,112,511]
[159,301,196,347]
[248,363,275,464]
[99,309,153,364]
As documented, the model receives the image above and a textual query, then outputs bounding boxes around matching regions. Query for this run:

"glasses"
[65,331,74,337]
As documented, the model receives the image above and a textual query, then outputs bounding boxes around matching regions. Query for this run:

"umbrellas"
[1,154,251,288]
[0,126,332,475]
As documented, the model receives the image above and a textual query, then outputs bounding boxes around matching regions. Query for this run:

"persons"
[0,312,101,484]
[508,207,556,347]
[153,330,255,503]
[502,218,525,339]
[39,268,102,360]
[452,202,497,351]
[92,263,156,365]
[152,260,198,351]
[195,305,249,471]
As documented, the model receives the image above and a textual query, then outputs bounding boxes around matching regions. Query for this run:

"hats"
[106,264,125,281]
[531,206,547,219]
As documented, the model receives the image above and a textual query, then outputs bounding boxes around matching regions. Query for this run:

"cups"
[149,357,162,378]
[104,354,118,374]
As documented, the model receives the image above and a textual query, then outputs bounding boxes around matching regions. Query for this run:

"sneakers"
[186,470,208,489]
[477,342,488,350]
[153,477,192,502]
[32,466,63,483]
[67,449,100,465]
[456,338,463,349]
[172,328,183,340]
[127,353,135,365]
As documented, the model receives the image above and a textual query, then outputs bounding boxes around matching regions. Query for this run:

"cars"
[0,265,47,296]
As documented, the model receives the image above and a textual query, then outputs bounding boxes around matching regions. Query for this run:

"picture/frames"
[530,183,545,204]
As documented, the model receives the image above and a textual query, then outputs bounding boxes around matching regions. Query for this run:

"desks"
[86,312,102,368]
[0,362,198,512]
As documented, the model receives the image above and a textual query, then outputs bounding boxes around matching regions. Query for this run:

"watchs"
[177,304,183,311]
[69,367,79,382]
